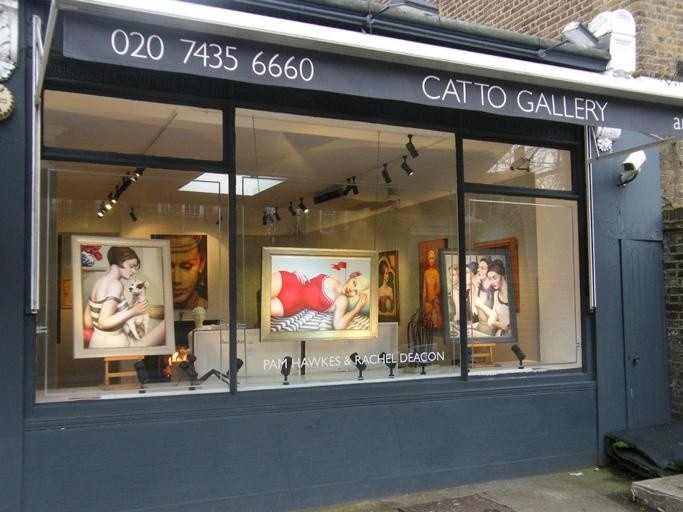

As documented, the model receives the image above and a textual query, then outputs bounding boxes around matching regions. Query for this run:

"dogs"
[122,280,148,341]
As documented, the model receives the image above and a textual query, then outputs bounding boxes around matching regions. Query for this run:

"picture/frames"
[260,237,521,345]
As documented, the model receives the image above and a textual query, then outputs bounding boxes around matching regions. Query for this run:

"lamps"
[95,166,146,218]
[262,133,419,226]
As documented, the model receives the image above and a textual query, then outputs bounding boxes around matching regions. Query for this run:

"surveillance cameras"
[510,158,525,171]
[621,150,647,173]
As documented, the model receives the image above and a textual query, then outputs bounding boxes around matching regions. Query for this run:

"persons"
[422,248,443,329]
[270,268,371,330]
[163,235,208,311]
[466,257,510,338]
[378,261,394,313]
[447,264,461,322]
[82,246,166,349]
